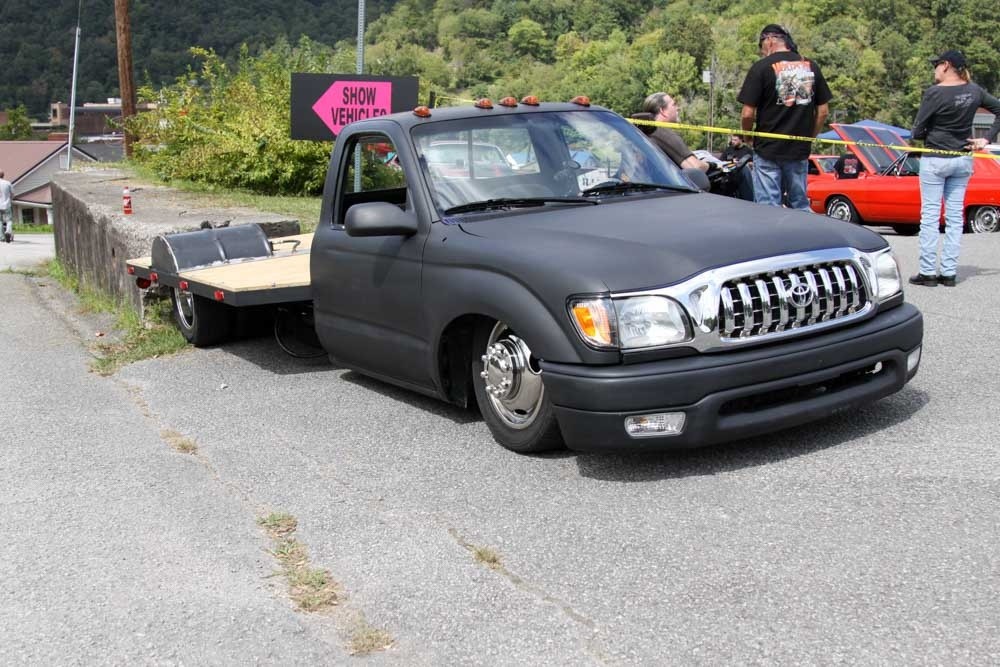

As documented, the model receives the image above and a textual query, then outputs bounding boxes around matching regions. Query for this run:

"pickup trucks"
[122,94,927,460]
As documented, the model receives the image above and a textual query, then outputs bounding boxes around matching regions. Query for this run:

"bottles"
[123,187,132,215]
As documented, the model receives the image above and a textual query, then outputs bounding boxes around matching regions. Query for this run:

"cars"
[806,123,1000,238]
[382,141,513,179]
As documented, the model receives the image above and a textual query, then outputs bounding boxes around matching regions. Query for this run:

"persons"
[737,24,834,211]
[908,50,1000,286]
[719,134,753,168]
[0,170,15,243]
[644,93,717,175]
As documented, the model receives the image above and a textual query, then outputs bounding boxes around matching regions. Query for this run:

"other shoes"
[5,232,11,243]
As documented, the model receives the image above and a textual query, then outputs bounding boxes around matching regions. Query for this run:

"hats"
[928,48,965,65]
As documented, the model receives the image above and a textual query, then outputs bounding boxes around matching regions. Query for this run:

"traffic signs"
[289,70,420,142]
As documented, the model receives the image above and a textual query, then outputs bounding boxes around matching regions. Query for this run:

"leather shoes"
[909,272,938,287]
[936,274,957,286]
